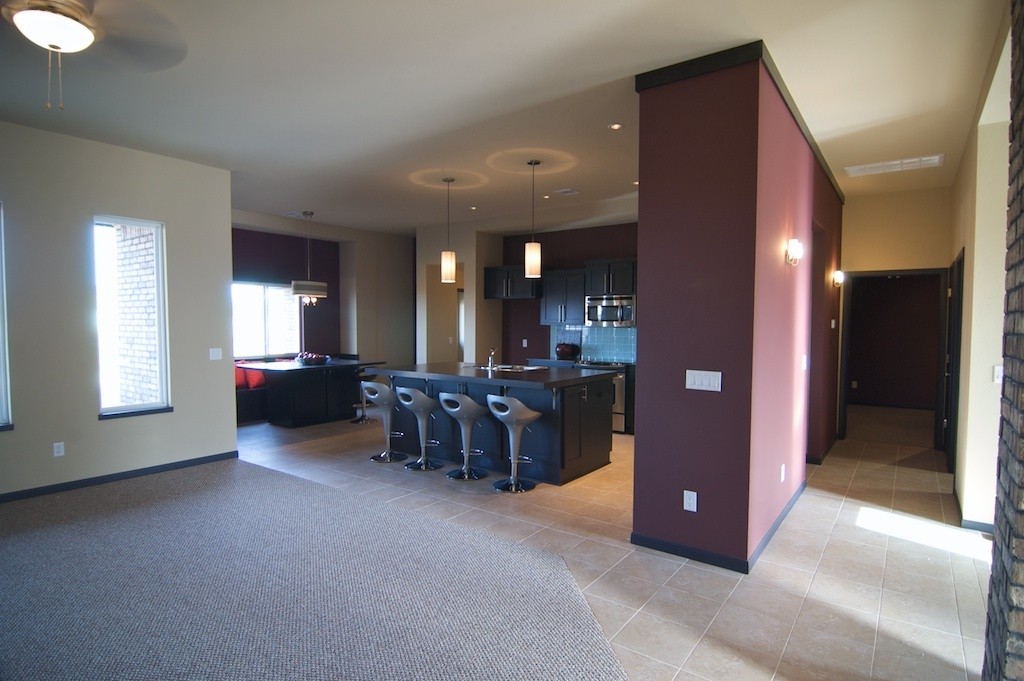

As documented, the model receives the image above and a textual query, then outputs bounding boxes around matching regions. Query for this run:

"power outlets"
[53,442,64,457]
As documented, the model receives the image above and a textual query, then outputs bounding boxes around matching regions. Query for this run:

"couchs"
[234,356,297,424]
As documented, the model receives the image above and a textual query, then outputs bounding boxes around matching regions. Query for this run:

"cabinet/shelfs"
[484,265,543,299]
[585,257,636,296]
[561,378,614,469]
[540,269,585,325]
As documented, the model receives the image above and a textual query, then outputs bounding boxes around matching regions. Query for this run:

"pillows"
[234,359,266,388]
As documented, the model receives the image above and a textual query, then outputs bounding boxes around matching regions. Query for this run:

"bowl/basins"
[296,357,328,365]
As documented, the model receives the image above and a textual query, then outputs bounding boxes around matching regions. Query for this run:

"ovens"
[611,372,628,432]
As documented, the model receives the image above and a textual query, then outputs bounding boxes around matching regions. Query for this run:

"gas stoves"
[573,360,631,369]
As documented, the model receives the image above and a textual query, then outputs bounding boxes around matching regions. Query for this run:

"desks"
[237,360,386,429]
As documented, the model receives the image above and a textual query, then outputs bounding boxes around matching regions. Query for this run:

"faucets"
[488,347,495,368]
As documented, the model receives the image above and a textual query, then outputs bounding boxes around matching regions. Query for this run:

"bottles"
[556,342,572,359]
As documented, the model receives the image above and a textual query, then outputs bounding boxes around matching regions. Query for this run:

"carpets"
[0,458,631,681]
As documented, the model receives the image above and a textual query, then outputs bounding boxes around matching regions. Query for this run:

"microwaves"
[584,295,636,328]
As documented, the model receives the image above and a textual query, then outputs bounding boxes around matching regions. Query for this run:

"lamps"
[441,178,457,283]
[524,160,541,278]
[787,240,802,267]
[292,211,328,307]
[833,269,844,287]
[13,9,96,108]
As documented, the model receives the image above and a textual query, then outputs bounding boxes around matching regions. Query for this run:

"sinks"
[480,365,549,373]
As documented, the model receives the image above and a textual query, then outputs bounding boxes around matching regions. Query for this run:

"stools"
[396,386,444,471]
[350,366,377,424]
[361,381,408,463]
[488,394,543,493]
[439,392,488,480]
